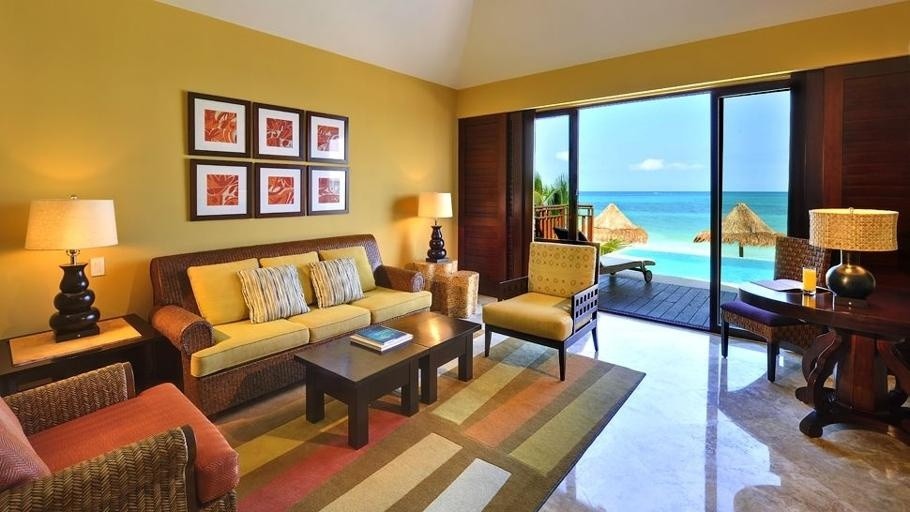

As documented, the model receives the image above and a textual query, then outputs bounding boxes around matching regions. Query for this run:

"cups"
[803,267,816,295]
[801,295,815,310]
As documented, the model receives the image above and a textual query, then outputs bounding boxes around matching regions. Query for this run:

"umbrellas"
[590,204,648,246]
[693,203,784,257]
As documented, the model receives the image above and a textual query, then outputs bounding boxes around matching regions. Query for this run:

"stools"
[432,269,480,317]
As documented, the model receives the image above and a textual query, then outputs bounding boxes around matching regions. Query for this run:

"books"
[750,278,834,292]
[350,323,413,353]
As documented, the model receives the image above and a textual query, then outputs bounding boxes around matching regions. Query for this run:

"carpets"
[180,327,646,511]
[592,273,770,342]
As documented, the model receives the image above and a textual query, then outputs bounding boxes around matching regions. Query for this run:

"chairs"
[0,361,240,510]
[482,237,602,380]
[718,233,840,384]
[553,226,657,288]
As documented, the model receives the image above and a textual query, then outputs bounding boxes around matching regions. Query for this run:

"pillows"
[306,253,365,310]
[237,264,312,323]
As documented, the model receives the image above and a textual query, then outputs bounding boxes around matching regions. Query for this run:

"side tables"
[1,312,169,398]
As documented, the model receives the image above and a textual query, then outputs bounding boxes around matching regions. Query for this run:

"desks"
[739,280,910,440]
[404,257,458,291]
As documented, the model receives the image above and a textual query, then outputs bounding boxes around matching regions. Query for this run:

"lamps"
[805,206,901,300]
[416,192,452,264]
[23,194,120,343]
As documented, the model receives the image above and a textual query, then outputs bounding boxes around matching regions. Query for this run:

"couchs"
[149,232,434,417]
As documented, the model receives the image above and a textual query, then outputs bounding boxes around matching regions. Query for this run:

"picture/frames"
[251,102,307,161]
[306,108,348,164]
[251,159,306,218]
[189,154,252,222]
[186,90,252,158]
[306,164,351,216]
